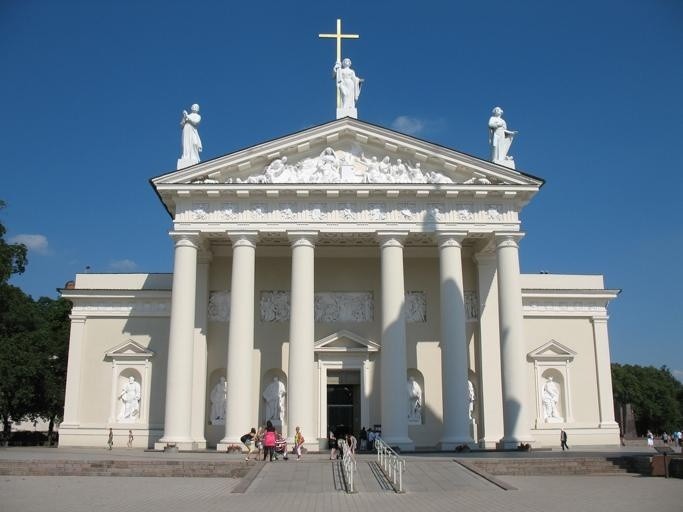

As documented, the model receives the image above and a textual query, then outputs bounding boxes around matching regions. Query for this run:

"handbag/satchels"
[246,440,251,445]
[241,434,248,442]
[298,437,304,445]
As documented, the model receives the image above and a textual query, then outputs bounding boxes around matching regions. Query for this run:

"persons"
[263,374,286,428]
[241,428,256,461]
[179,103,202,161]
[543,376,560,418]
[560,427,568,451]
[329,425,380,460]
[240,147,491,185]
[127,431,133,448]
[108,428,113,450]
[206,290,479,324]
[646,430,653,450]
[468,379,475,419]
[408,375,422,426]
[255,420,288,462]
[295,427,304,460]
[333,58,364,118]
[118,375,141,421]
[210,374,227,426]
[489,105,517,163]
[663,431,683,447]
